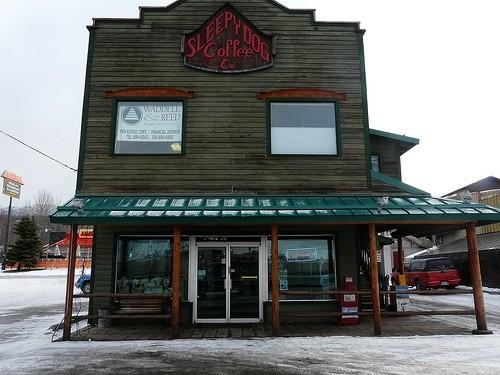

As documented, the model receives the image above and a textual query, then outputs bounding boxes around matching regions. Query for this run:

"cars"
[75,274,91,294]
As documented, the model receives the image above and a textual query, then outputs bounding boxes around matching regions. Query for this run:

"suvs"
[404,253,462,290]
